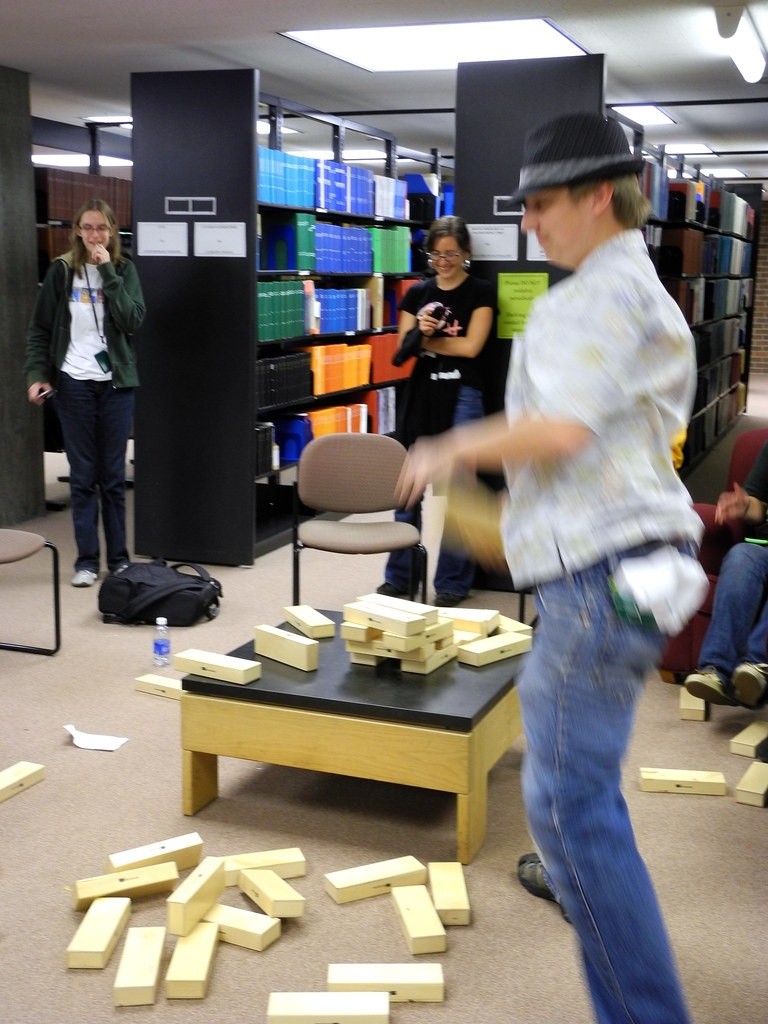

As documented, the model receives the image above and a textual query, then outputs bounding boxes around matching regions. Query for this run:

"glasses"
[77,224,107,232]
[428,251,464,261]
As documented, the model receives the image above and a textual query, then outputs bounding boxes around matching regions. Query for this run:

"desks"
[180,608,537,866]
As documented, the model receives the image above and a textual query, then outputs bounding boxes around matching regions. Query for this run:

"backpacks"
[99,556,223,625]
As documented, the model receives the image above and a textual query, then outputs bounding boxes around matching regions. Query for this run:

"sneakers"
[518,852,572,923]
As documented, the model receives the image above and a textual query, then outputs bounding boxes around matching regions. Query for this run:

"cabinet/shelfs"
[0,53,763,593]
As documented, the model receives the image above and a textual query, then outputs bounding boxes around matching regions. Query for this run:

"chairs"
[0,528,63,656]
[293,432,429,605]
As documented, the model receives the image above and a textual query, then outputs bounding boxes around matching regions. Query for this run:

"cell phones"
[37,390,52,398]
[428,306,447,325]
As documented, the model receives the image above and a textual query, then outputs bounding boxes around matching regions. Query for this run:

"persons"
[375,212,501,608]
[394,106,708,1022]
[682,442,768,708]
[18,198,150,586]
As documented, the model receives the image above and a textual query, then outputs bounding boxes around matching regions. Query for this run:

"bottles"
[154,617,170,665]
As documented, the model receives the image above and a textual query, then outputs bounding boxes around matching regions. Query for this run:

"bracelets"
[743,499,750,519]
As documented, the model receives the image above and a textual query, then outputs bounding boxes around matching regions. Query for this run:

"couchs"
[650,428,768,685]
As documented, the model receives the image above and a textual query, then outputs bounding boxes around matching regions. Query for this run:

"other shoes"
[732,662,768,706]
[71,570,98,587]
[684,666,738,706]
[112,565,128,577]
[377,582,419,596]
[436,592,464,606]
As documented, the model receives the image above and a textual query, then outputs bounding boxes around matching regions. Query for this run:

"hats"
[504,111,644,209]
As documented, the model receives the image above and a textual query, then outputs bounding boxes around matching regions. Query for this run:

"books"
[637,158,755,462]
[255,145,442,474]
